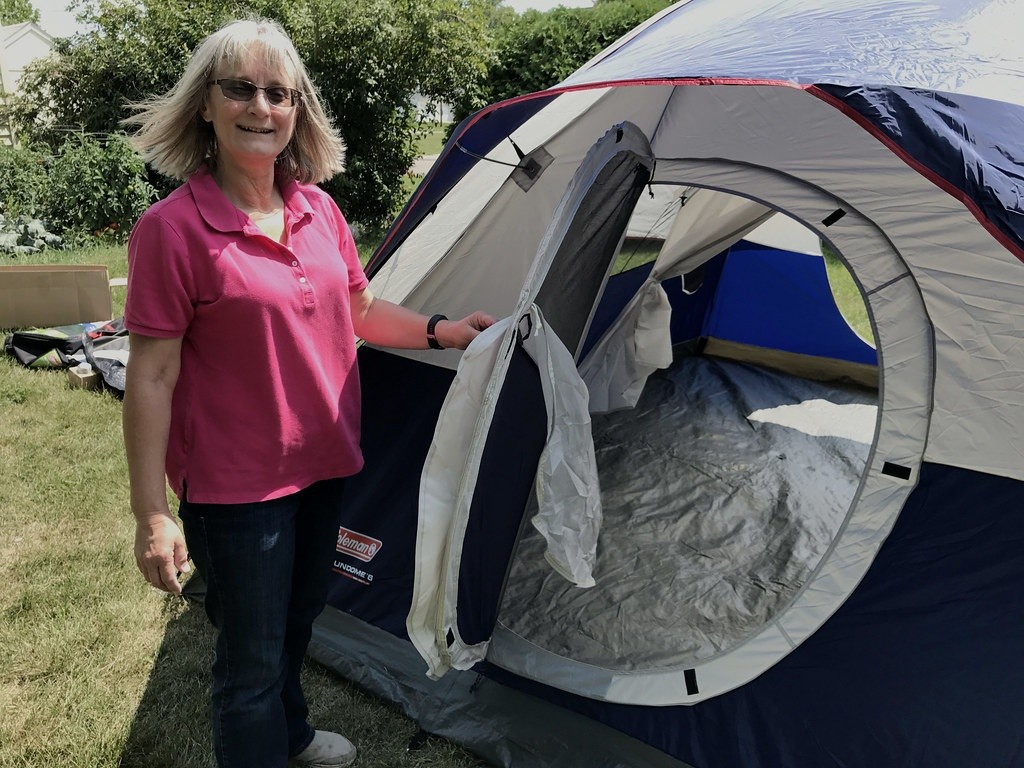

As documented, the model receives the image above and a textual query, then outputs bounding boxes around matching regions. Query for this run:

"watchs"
[427,314,448,350]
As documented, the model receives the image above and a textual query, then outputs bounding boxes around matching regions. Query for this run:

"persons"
[122,18,499,767]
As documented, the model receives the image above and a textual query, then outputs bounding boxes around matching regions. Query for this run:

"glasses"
[208,78,302,108]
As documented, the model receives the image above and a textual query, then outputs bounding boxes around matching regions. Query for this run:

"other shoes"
[297,729,358,767]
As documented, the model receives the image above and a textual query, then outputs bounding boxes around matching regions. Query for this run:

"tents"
[164,0,1024,767]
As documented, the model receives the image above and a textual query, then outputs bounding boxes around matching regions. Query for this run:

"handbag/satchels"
[82,316,130,392]
[6,321,108,368]
